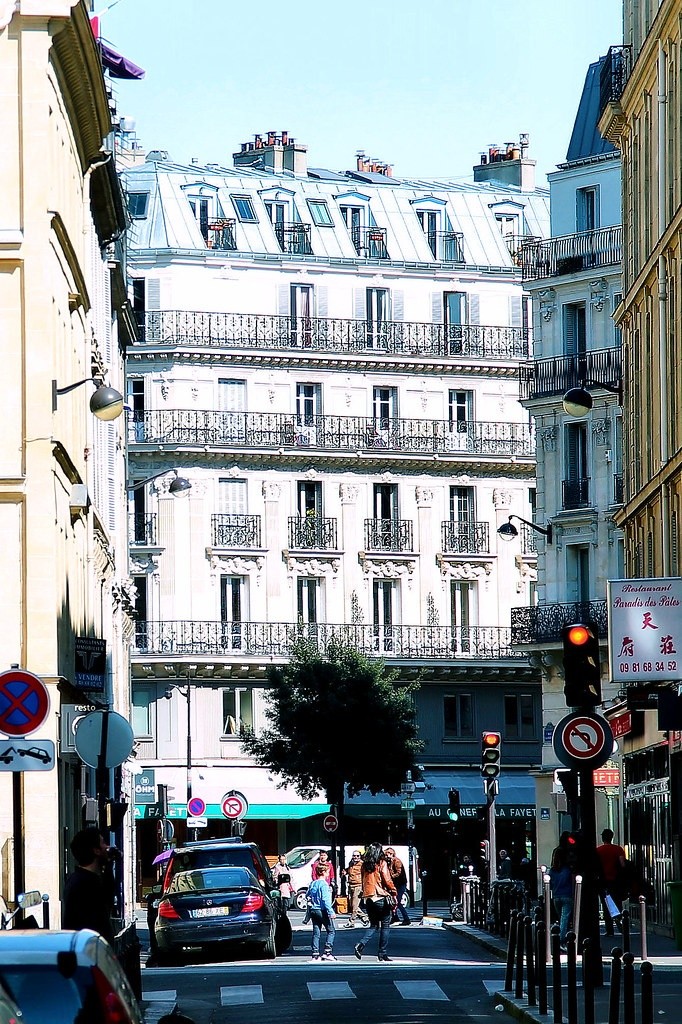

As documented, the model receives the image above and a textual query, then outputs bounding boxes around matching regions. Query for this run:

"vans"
[271,845,423,908]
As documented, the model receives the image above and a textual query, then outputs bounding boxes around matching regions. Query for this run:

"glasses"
[353,854,361,857]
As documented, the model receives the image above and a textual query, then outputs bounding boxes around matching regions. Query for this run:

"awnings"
[343,775,536,804]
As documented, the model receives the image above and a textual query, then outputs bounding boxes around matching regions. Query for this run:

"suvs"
[152,837,292,952]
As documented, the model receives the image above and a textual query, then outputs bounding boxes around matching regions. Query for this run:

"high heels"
[378,953,392,961]
[353,943,363,959]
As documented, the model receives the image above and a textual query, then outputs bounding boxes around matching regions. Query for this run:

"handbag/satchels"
[603,895,621,918]
[386,894,398,909]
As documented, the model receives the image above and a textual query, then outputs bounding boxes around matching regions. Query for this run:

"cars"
[154,869,280,966]
[0,926,143,1024]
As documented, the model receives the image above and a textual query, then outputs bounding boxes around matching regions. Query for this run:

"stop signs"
[323,815,338,832]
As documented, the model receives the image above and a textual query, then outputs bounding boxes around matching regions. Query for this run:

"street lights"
[166,683,192,842]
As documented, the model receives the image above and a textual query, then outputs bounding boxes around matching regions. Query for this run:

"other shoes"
[391,916,400,924]
[606,931,614,936]
[560,943,569,951]
[400,919,411,925]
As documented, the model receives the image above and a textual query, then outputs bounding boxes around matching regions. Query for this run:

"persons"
[353,842,398,961]
[497,849,515,879]
[104,847,123,919]
[551,831,574,872]
[312,850,335,903]
[550,848,573,950]
[520,854,532,893]
[384,847,411,925]
[274,854,297,913]
[61,826,114,951]
[597,828,626,936]
[342,850,369,928]
[305,863,338,961]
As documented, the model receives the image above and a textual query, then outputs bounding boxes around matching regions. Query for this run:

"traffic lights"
[563,624,600,708]
[157,784,175,815]
[479,839,489,861]
[560,830,580,874]
[481,733,501,777]
[448,792,460,822]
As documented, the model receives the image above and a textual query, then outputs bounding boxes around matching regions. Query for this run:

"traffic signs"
[401,800,426,812]
[400,782,426,793]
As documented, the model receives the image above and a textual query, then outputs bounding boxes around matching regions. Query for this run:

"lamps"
[497,514,553,546]
[562,378,624,419]
[51,377,124,421]
[125,467,193,498]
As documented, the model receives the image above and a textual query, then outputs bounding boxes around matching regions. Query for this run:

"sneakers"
[362,921,369,928]
[312,956,319,962]
[343,920,355,929]
[321,953,337,962]
